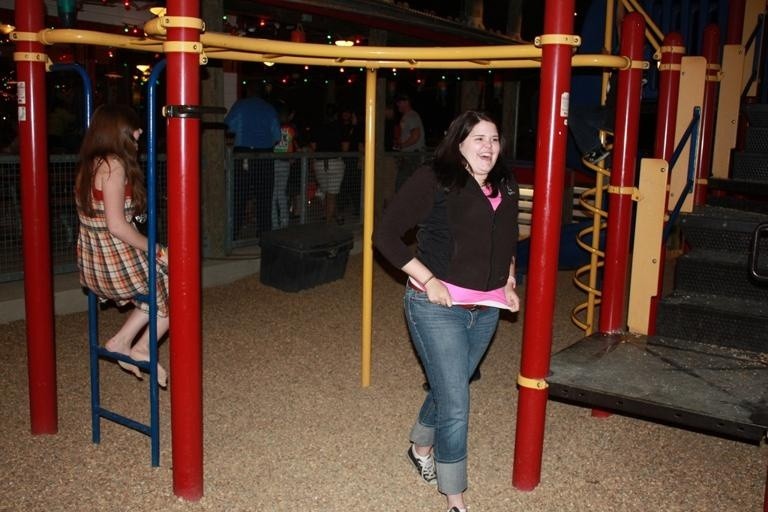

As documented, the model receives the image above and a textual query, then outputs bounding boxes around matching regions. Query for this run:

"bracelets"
[421,274,436,288]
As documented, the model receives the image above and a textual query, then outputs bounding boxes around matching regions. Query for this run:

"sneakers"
[407,445,437,485]
[588,147,609,165]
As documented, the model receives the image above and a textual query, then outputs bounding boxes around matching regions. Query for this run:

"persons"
[74,102,169,390]
[225,79,283,242]
[269,91,427,234]
[370,108,521,512]
